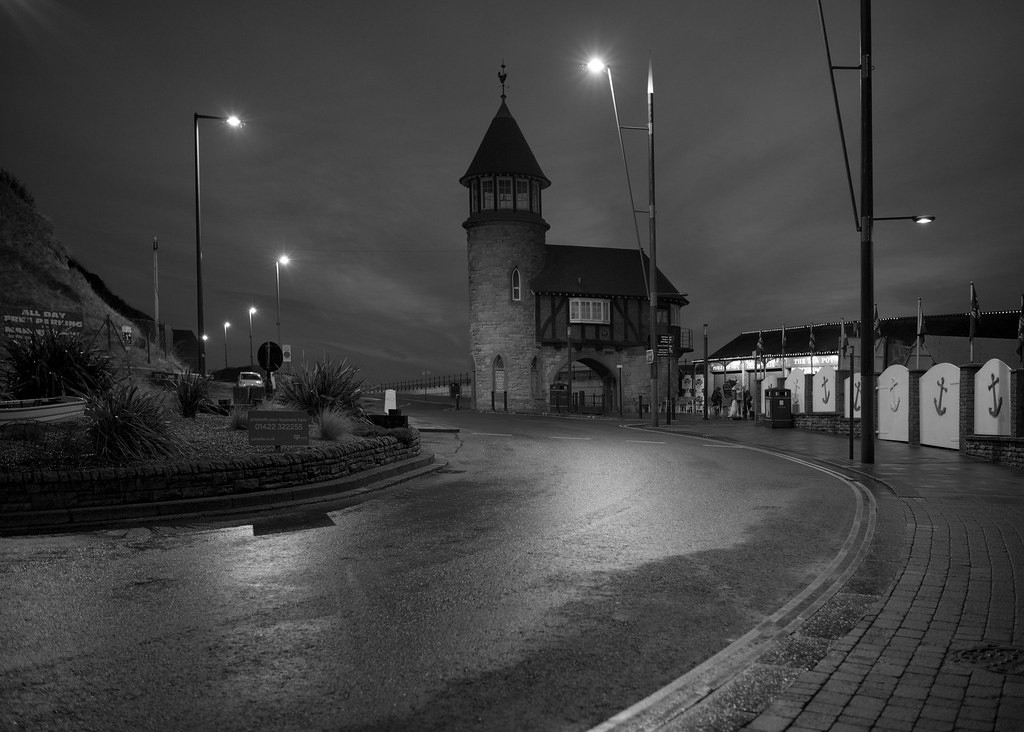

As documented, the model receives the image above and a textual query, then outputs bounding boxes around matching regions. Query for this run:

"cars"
[236,372,263,389]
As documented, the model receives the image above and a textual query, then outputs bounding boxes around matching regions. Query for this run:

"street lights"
[276,257,291,346]
[195,113,245,377]
[859,215,937,463]
[223,323,231,371]
[587,58,658,426]
[249,307,258,370]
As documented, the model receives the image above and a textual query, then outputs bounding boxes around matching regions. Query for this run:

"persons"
[711,387,722,413]
[744,391,753,415]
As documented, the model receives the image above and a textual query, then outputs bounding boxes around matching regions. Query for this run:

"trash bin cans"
[762,386,795,430]
[549,382,570,415]
[448,382,462,399]
